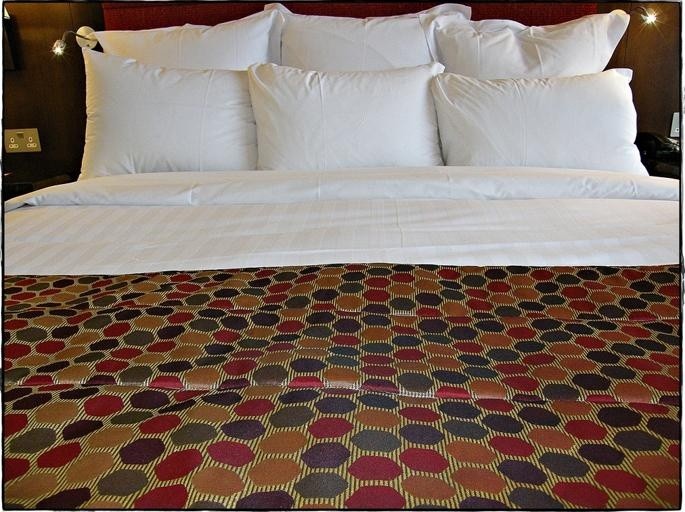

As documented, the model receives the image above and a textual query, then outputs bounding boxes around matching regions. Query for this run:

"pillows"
[76,4,647,178]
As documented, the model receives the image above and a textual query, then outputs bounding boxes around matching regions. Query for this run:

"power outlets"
[3,128,41,155]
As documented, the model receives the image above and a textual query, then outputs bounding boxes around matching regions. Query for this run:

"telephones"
[638,132,680,159]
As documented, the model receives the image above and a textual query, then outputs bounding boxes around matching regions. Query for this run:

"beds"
[3,154,683,508]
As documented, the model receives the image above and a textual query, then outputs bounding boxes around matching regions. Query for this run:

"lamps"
[50,28,97,59]
